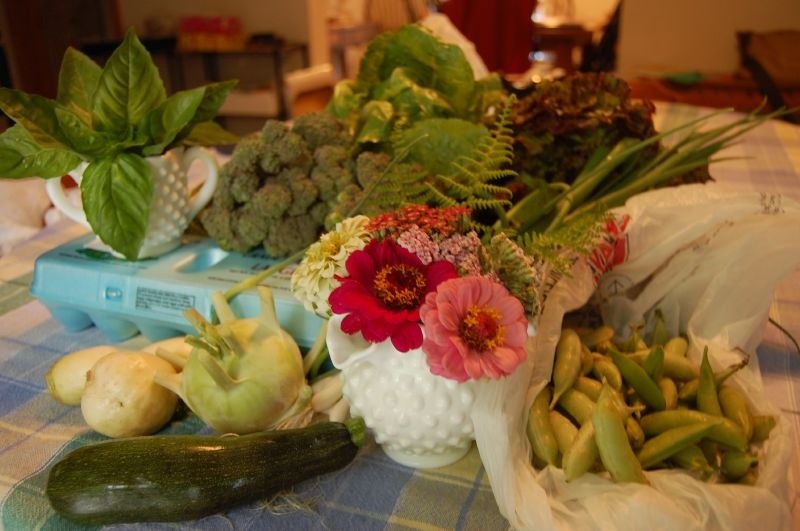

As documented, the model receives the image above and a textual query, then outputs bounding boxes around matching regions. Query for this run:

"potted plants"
[0,26,243,261]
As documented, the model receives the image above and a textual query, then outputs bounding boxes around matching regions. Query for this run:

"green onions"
[281,100,800,429]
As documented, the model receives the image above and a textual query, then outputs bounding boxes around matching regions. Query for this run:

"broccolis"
[197,109,396,258]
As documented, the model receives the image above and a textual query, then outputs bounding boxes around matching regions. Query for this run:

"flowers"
[290,203,538,384]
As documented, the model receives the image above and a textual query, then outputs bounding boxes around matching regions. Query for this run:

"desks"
[77,32,275,152]
[0,98,800,531]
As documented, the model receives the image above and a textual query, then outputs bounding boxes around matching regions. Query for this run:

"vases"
[326,313,537,470]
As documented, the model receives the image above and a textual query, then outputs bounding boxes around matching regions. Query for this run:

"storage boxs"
[30,232,336,376]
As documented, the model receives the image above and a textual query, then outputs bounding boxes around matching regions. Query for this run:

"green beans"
[520,317,771,487]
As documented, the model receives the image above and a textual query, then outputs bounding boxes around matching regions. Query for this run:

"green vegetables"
[326,24,716,215]
[0,28,244,262]
